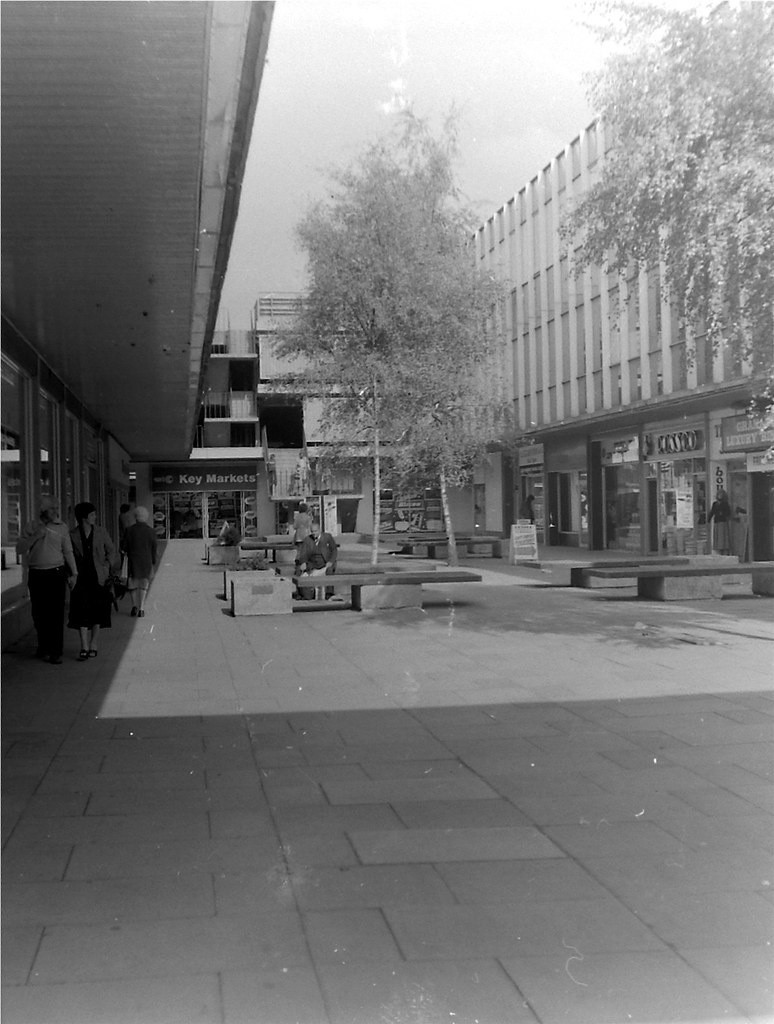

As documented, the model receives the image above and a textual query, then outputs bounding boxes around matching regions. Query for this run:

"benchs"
[396,538,502,560]
[241,541,343,564]
[275,562,482,611]
[524,556,773,601]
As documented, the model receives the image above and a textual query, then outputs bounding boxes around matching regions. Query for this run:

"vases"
[231,578,292,615]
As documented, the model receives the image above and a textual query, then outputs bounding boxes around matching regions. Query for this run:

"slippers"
[89,650,97,657]
[79,649,89,659]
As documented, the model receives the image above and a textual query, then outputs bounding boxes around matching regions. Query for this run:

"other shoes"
[325,593,334,599]
[36,651,50,662]
[131,606,138,616]
[50,654,63,663]
[138,610,144,616]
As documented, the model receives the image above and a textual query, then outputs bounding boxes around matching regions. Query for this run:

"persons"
[65,502,121,661]
[17,495,78,665]
[706,490,732,555]
[266,453,277,486]
[117,503,157,617]
[290,452,310,496]
[294,503,337,600]
[518,494,534,524]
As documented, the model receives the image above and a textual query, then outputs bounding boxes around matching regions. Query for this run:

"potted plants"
[206,527,243,564]
[223,554,275,601]
